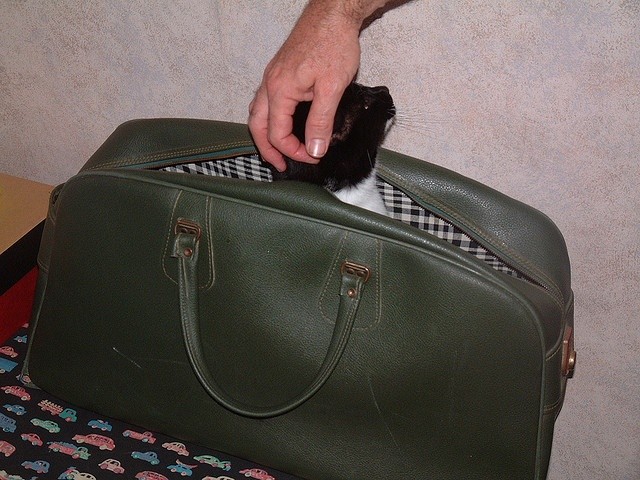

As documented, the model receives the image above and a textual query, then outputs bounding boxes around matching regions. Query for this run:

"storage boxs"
[1,173,52,344]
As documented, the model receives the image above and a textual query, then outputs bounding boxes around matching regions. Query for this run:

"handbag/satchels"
[19,120,573,479]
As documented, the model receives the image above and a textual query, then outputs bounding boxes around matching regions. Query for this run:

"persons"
[248,0,390,173]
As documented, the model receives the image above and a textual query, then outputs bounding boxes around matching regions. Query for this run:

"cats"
[261,81,403,217]
